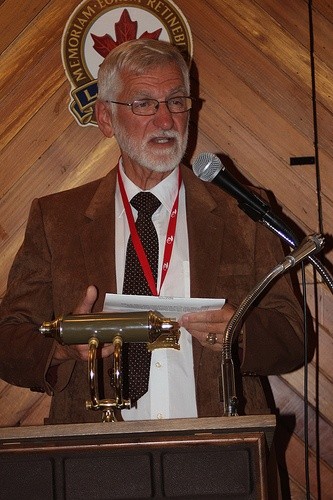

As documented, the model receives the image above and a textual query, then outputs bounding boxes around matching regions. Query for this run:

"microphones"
[192,152,298,250]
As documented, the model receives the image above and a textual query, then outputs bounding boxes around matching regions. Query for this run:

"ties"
[122,191,162,296]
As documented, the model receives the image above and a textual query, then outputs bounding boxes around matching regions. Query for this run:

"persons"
[0,39,306,419]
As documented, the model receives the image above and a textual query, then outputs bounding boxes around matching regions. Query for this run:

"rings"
[204,333,218,345]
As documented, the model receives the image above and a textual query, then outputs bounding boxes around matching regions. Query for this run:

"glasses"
[104,96,196,116]
